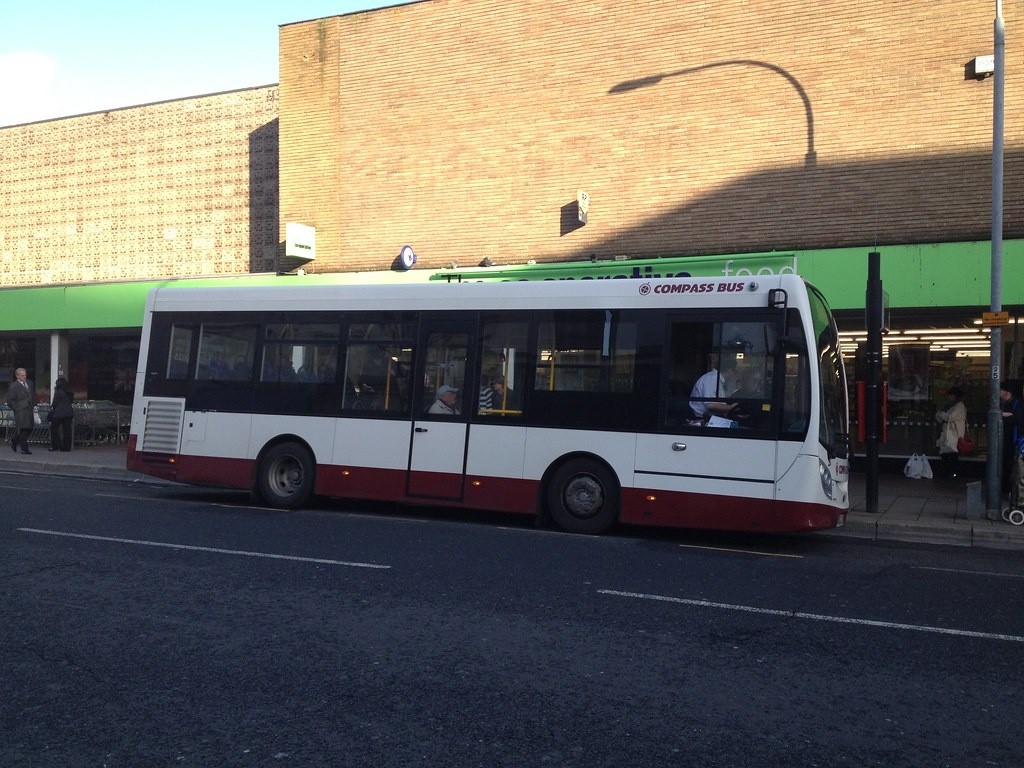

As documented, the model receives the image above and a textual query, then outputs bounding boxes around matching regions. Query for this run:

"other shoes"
[49,447,60,451]
[10,439,17,452]
[60,448,70,451]
[21,450,32,455]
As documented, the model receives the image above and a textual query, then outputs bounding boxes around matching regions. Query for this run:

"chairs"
[233,355,250,380]
[280,366,298,383]
[298,366,316,384]
[318,364,335,383]
[261,364,278,382]
[211,351,230,382]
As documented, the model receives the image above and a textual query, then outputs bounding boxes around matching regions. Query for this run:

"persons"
[48,378,74,452]
[6,368,38,455]
[998,381,1022,500]
[935,385,967,479]
[425,356,762,421]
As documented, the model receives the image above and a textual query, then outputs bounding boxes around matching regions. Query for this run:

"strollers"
[1002,453,1024,526]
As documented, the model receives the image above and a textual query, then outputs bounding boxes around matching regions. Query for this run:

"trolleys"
[1,401,135,445]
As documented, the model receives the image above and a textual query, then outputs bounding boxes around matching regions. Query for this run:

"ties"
[23,382,28,388]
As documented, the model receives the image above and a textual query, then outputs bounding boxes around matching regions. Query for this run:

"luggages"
[1002,440,1024,526]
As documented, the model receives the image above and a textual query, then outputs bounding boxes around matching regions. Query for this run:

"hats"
[490,375,504,383]
[717,358,738,373]
[1000,381,1017,393]
[436,385,459,397]
[946,387,964,397]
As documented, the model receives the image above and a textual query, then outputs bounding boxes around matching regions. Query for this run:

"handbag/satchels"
[33,408,41,426]
[1014,436,1024,457]
[921,455,934,480]
[904,453,923,479]
[957,435,975,456]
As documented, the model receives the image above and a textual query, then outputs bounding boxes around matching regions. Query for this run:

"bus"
[126,275,856,535]
